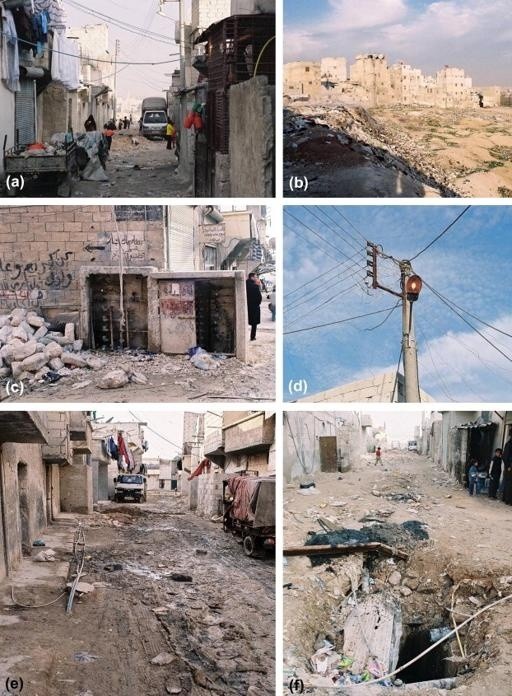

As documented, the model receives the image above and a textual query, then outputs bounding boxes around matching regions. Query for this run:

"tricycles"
[222,476,275,557]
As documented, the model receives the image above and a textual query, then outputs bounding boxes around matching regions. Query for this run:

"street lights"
[400,273,428,351]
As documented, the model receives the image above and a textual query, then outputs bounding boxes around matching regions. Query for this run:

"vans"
[114,474,147,503]
[140,111,168,140]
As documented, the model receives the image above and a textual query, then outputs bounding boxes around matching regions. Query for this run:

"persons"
[171,122,178,148]
[500,428,512,506]
[488,448,502,499]
[468,458,478,495]
[129,133,139,146]
[105,127,115,150]
[267,285,277,322]
[103,114,134,130]
[166,119,174,150]
[245,272,263,342]
[373,447,384,466]
